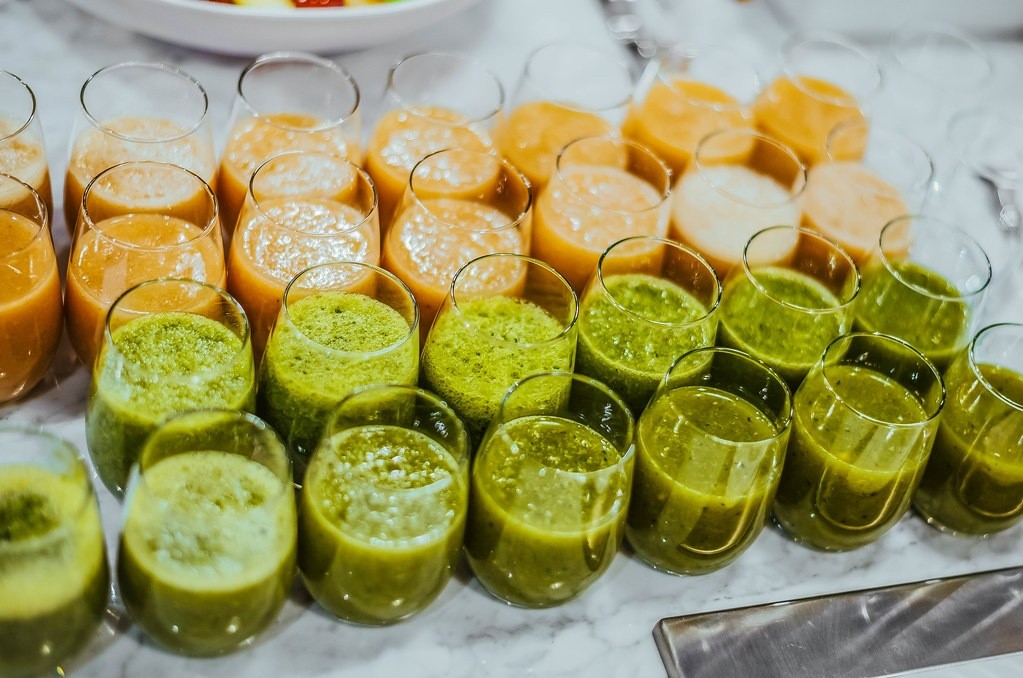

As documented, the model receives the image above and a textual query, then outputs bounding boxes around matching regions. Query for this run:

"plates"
[64,0,489,59]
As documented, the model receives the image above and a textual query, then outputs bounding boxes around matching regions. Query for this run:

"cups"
[116,405,301,660]
[0,422,110,678]
[470,367,639,610]
[59,32,995,495]
[0,169,65,403]
[0,69,53,232]
[298,381,472,625]
[622,347,794,577]
[911,321,1023,538]
[773,330,948,555]
